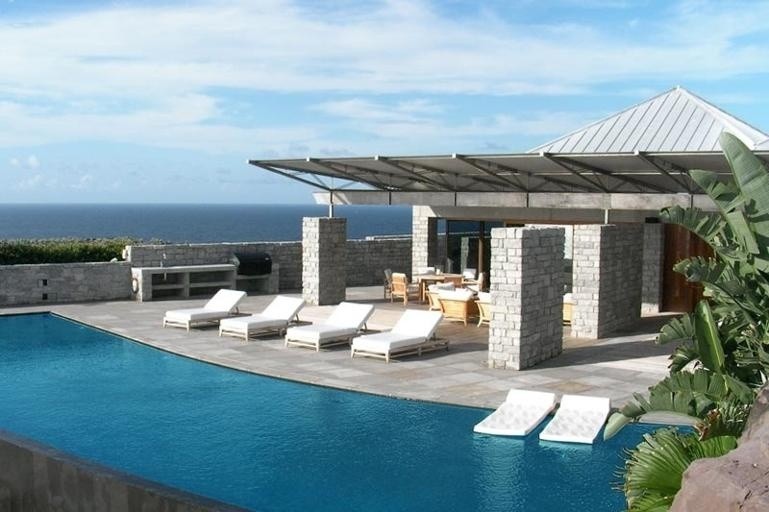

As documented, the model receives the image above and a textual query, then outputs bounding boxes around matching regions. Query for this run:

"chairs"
[562,292,572,325]
[384,267,490,327]
[163,289,448,363]
